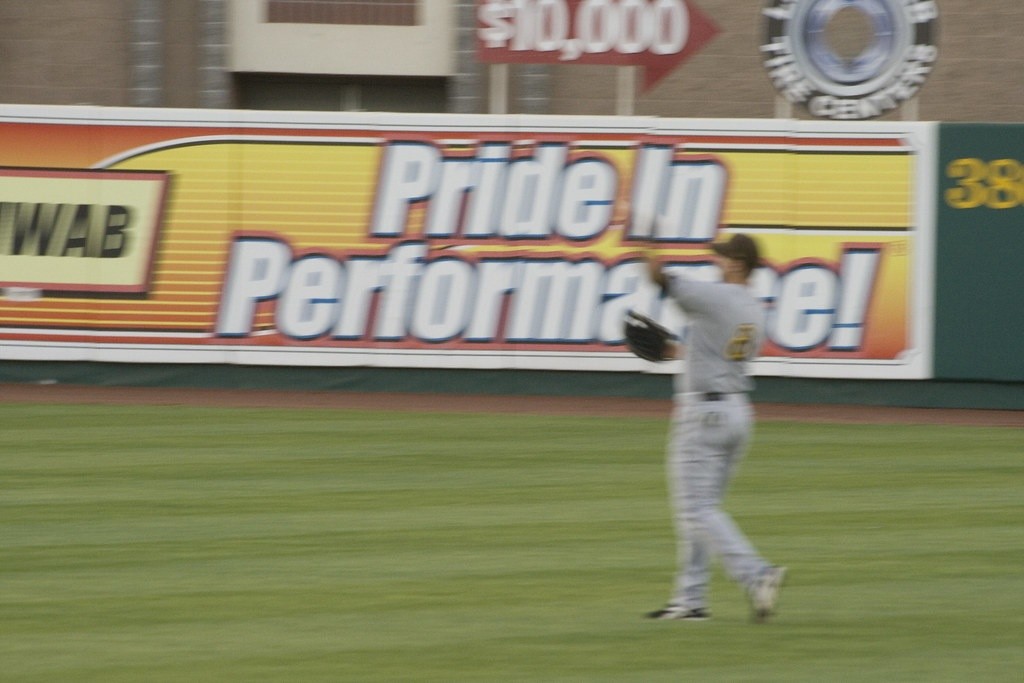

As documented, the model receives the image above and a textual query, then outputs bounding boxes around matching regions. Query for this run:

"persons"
[643,234,788,625]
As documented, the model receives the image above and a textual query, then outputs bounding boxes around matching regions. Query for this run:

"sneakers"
[752,565,789,619]
[643,604,712,622]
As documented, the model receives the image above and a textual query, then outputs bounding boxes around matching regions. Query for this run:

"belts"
[700,392,726,401]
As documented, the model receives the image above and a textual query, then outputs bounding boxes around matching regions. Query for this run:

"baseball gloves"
[621,307,683,363]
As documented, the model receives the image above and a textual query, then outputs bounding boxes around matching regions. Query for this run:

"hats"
[709,233,768,267]
